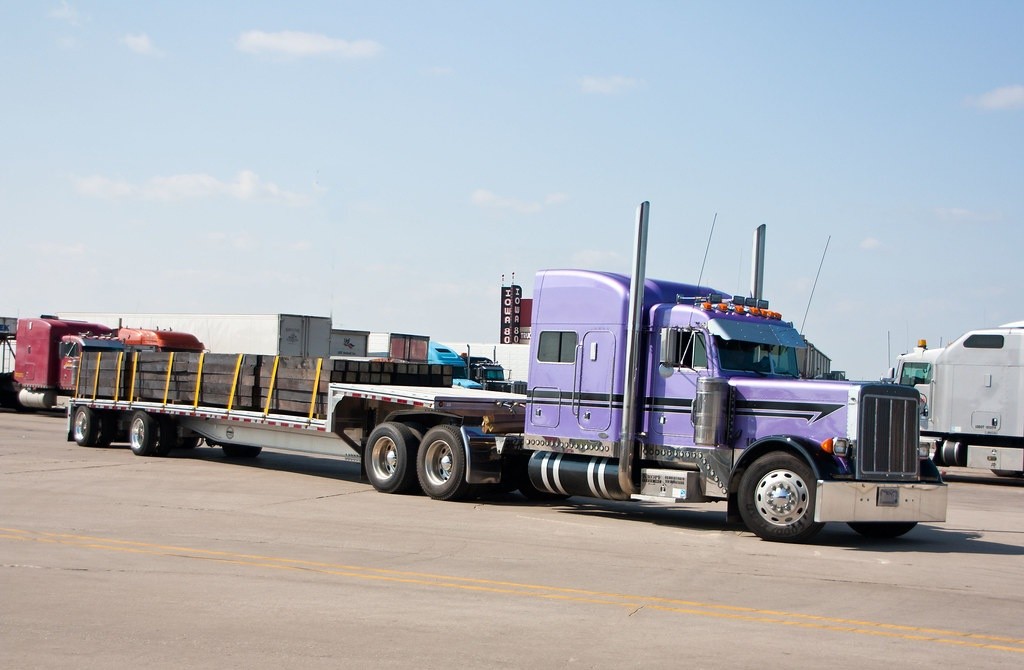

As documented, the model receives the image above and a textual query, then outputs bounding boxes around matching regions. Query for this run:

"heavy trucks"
[0,314,528,414]
[887,321,1024,482]
[68,200,949,541]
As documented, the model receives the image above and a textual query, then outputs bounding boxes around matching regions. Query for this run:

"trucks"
[427,343,506,390]
